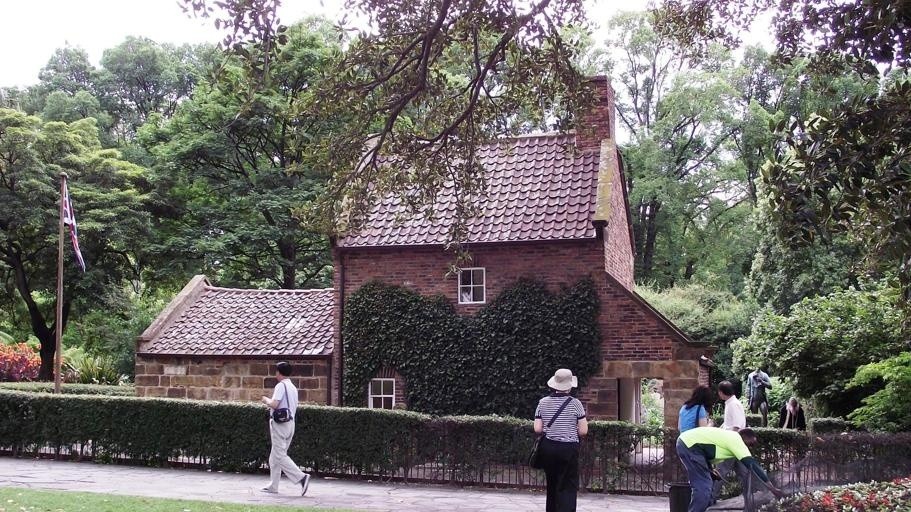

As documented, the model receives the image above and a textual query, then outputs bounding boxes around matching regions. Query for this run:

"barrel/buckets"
[664,481,691,512]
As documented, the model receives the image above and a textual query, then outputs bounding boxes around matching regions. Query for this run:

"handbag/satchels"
[274,408,291,422]
[528,436,544,469]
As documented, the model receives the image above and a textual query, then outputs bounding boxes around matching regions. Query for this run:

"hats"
[276,362,292,376]
[547,369,578,391]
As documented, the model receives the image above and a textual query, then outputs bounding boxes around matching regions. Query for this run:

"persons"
[717,381,754,512]
[778,397,806,487]
[746,367,772,428]
[675,426,783,512]
[678,385,714,434]
[534,369,589,512]
[261,362,311,496]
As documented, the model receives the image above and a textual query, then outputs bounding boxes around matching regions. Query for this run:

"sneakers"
[262,487,270,492]
[301,474,311,496]
